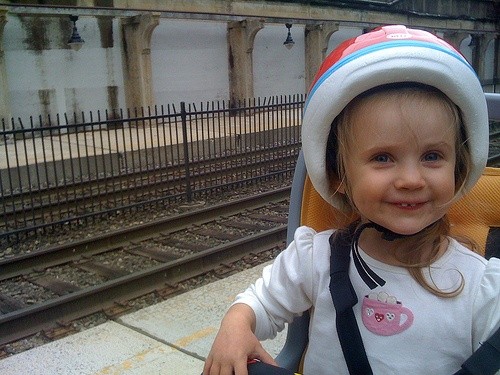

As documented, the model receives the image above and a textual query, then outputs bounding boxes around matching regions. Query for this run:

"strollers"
[200,93,500,375]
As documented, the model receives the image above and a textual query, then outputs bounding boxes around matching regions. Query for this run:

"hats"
[300,22,489,212]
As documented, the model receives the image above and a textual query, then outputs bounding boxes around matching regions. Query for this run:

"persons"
[200,26,500,375]
